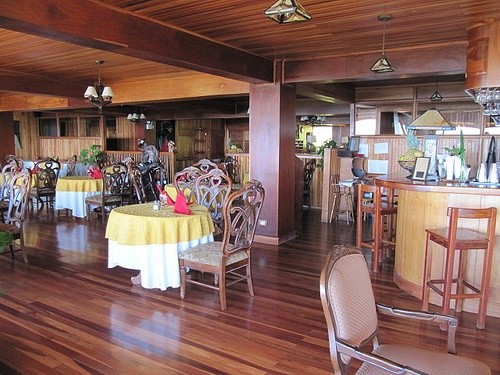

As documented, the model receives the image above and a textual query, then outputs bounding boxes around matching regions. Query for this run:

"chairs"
[303,159,316,213]
[0,154,265,311]
[319,242,491,375]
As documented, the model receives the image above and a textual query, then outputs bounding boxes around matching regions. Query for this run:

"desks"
[0,172,48,200]
[164,183,232,215]
[54,176,110,218]
[105,201,216,291]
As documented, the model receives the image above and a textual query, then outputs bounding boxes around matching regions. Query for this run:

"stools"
[328,175,398,273]
[421,207,497,333]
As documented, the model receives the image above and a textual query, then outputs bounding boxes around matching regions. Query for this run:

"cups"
[478,163,487,187]
[488,163,500,188]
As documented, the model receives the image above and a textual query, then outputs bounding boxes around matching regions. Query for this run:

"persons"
[137,136,157,177]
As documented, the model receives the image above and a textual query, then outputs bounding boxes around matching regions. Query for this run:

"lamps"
[83,60,114,110]
[430,76,443,101]
[370,15,396,73]
[299,116,327,125]
[406,104,456,130]
[263,0,312,24]
[127,113,146,122]
